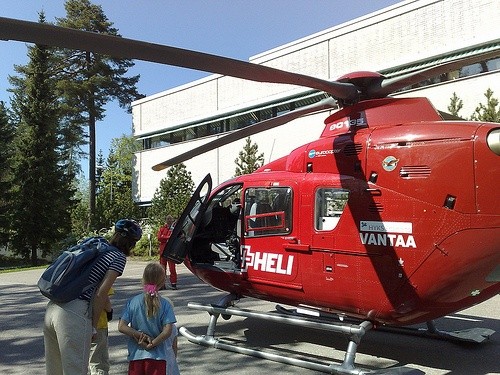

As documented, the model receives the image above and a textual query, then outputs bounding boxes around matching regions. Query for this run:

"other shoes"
[171,282,177,289]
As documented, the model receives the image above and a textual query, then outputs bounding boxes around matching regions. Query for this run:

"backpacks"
[38,232,121,308]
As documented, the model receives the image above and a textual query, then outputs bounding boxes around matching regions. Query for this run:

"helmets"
[115,216,143,242]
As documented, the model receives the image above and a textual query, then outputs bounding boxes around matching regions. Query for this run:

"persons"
[43,219,143,375]
[118,263,180,375]
[157,215,177,290]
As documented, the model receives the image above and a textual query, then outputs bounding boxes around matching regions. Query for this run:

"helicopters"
[0,14,500,375]
